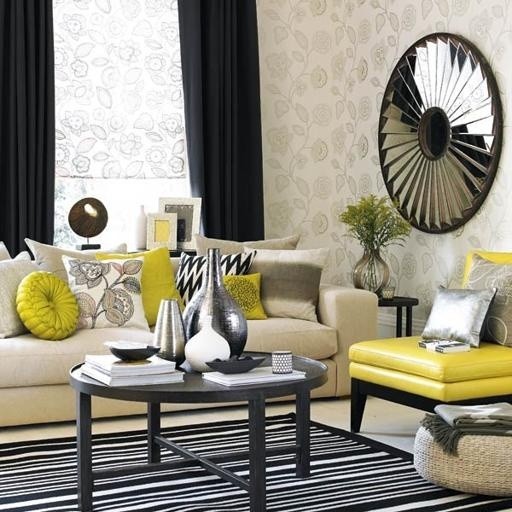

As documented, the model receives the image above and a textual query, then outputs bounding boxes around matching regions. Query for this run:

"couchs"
[347,247,512,436]
[0,244,368,425]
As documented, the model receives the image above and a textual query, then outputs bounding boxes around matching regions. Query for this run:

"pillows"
[193,233,302,258]
[94,247,184,327]
[243,246,330,322]
[61,254,151,331]
[0,250,42,338]
[464,253,512,347]
[421,283,497,350]
[15,270,79,341]
[221,271,267,320]
[175,251,257,305]
[23,238,127,282]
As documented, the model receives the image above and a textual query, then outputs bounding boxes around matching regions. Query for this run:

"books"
[81,354,185,386]
[201,366,306,386]
[418,339,471,354]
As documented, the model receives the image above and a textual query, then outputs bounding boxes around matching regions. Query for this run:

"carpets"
[0,410,512,512]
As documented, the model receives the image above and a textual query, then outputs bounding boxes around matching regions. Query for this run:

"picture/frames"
[158,197,201,250]
[146,213,177,250]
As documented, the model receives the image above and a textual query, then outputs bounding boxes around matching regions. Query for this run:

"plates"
[206,357,267,374]
[110,345,160,361]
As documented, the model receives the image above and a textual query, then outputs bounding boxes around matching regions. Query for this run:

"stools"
[412,423,512,497]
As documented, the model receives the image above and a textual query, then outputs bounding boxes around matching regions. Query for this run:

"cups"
[271,350,293,374]
[382,286,395,300]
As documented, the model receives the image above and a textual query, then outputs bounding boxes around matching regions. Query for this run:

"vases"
[182,247,248,357]
[184,316,231,372]
[134,203,147,250]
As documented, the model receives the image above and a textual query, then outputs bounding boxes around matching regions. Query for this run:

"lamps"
[69,198,107,249]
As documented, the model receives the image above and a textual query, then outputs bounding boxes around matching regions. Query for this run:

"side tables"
[375,296,418,338]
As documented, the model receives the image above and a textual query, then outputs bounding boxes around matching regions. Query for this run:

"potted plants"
[336,193,412,296]
